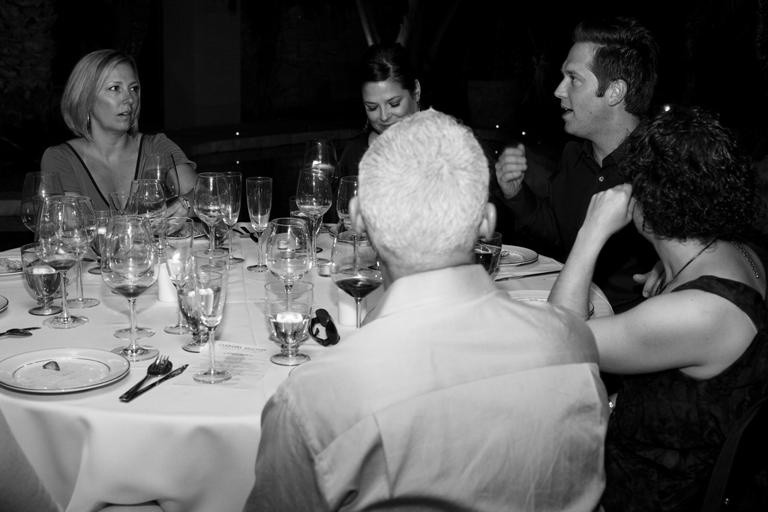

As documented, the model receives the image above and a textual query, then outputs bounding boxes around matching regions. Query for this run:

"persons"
[337,39,505,240]
[240,104,612,511]
[494,15,664,314]
[546,102,768,511]
[36,46,200,238]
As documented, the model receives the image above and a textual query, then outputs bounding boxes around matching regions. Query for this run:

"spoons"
[0,328,32,336]
[119,360,173,401]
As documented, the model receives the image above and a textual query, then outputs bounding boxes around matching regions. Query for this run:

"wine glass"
[471,233,503,282]
[20,139,384,384]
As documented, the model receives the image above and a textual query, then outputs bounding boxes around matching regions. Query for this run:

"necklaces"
[657,238,718,291]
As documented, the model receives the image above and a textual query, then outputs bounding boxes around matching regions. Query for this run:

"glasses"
[309,308,340,347]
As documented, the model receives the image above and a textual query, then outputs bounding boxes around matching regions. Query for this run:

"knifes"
[124,363,189,403]
[0,327,41,335]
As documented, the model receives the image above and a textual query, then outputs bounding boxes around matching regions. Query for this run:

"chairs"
[356,493,466,512]
[694,389,767,512]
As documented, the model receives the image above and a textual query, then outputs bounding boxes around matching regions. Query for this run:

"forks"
[122,355,169,402]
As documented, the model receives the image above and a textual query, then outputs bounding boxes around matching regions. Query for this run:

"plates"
[0,255,33,276]
[0,346,129,395]
[0,295,9,313]
[495,243,540,267]
[506,289,594,318]
